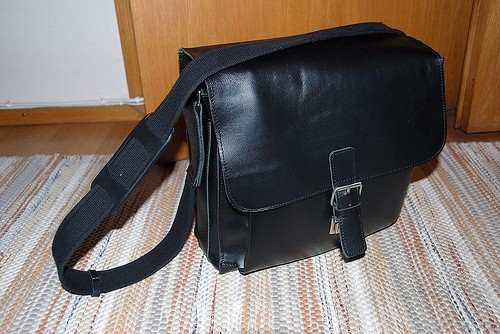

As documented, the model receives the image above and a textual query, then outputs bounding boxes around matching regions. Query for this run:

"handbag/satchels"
[52,22,447,296]
